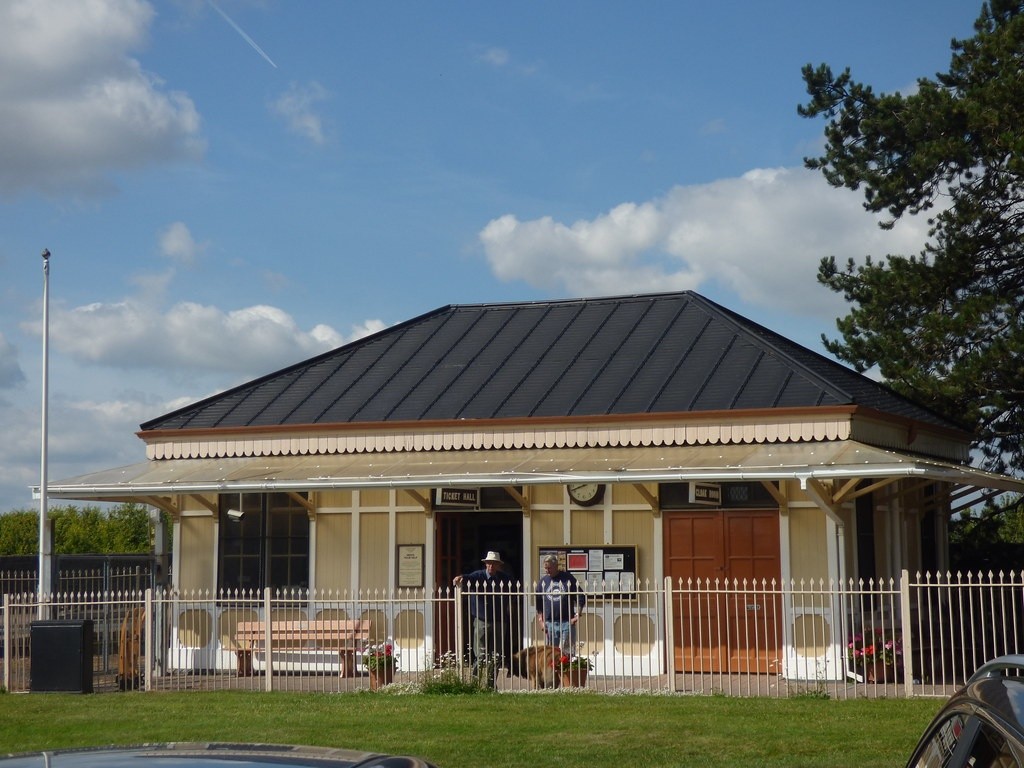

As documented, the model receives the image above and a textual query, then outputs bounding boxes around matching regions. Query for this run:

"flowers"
[847,627,903,663]
[550,641,594,672]
[362,644,398,671]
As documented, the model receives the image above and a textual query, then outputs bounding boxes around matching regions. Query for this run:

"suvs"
[903,653,1023,768]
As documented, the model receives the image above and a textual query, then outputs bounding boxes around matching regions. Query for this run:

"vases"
[558,669,587,687]
[859,658,895,684]
[369,670,392,689]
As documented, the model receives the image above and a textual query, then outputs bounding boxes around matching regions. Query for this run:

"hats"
[481,551,504,564]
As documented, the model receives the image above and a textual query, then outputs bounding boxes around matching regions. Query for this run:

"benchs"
[223,618,373,677]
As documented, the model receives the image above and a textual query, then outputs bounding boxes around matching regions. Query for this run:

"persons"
[536,555,586,656]
[453,551,511,689]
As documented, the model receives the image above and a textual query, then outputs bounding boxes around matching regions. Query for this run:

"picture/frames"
[397,544,423,588]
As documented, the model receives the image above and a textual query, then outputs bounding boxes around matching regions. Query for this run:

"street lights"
[38,247,51,622]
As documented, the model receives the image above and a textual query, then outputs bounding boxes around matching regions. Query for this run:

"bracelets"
[576,613,581,616]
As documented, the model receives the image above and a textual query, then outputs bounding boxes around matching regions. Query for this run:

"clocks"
[567,484,606,506]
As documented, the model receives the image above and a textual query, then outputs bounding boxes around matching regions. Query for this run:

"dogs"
[512,643,567,690]
